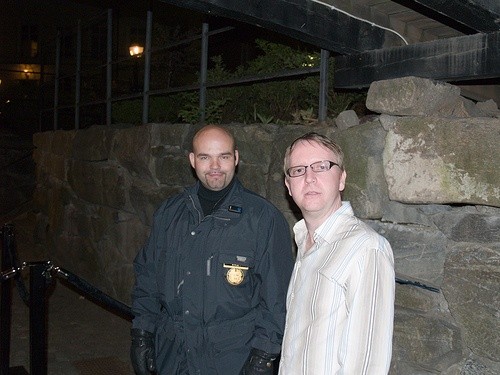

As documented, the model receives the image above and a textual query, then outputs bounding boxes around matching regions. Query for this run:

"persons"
[130,125,294,375]
[277,131,395,375]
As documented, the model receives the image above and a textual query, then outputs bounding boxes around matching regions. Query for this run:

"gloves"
[240,348,277,375]
[131,327,157,375]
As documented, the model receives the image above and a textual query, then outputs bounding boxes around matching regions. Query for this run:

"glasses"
[286,160,343,178]
